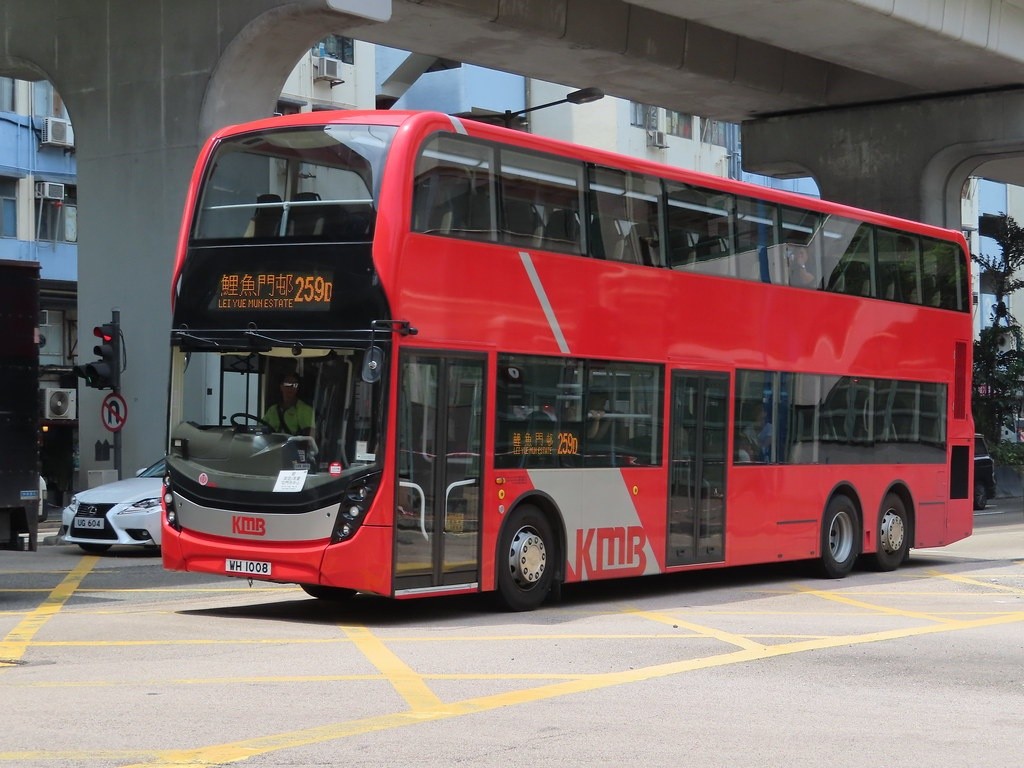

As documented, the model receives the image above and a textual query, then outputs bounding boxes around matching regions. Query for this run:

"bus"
[160,109,975,612]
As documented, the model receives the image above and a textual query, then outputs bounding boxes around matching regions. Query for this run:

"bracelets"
[802,265,805,268]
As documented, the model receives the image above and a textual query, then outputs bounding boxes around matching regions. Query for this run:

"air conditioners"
[43,385,78,422]
[653,134,677,154]
[38,182,65,200]
[319,56,345,84]
[44,120,75,148]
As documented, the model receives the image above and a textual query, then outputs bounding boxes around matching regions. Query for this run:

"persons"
[742,407,773,462]
[261,370,318,444]
[527,385,625,445]
[788,247,816,290]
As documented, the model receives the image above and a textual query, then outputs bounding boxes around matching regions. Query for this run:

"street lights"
[448,87,604,129]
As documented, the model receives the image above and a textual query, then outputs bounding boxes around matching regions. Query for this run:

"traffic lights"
[73,324,117,389]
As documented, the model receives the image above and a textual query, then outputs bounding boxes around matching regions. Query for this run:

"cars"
[57,458,166,553]
